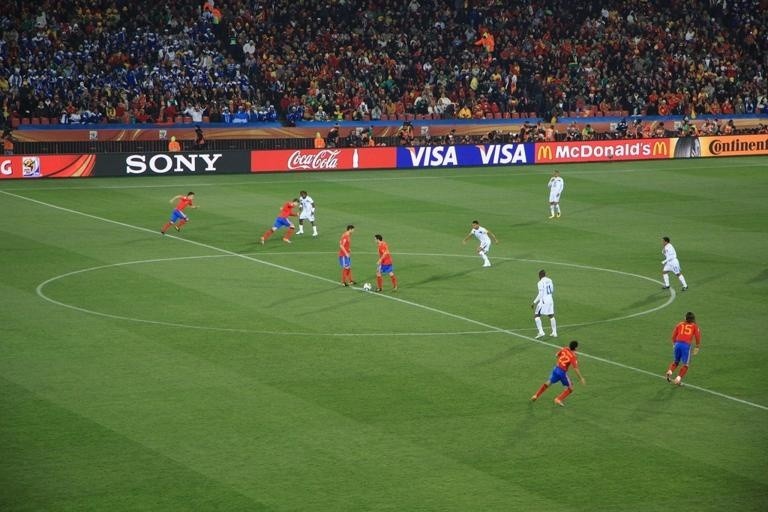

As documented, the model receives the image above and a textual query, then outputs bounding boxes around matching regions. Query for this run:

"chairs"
[343,110,630,122]
[11,115,210,128]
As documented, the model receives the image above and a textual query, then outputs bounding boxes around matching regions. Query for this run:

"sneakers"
[666,371,682,385]
[160,226,181,235]
[342,280,398,292]
[548,212,561,218]
[531,394,564,407]
[535,332,557,338]
[259,232,319,244]
[662,284,688,291]
[482,262,491,267]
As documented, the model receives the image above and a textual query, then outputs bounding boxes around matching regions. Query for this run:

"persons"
[338,226,356,286]
[296,191,319,237]
[660,237,688,290]
[531,340,585,406]
[160,192,200,235]
[547,170,564,218]
[374,234,397,291]
[259,198,300,244]
[463,221,499,267]
[531,270,558,339]
[666,312,701,386]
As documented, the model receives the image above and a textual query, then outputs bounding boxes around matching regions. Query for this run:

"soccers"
[363,283,371,290]
